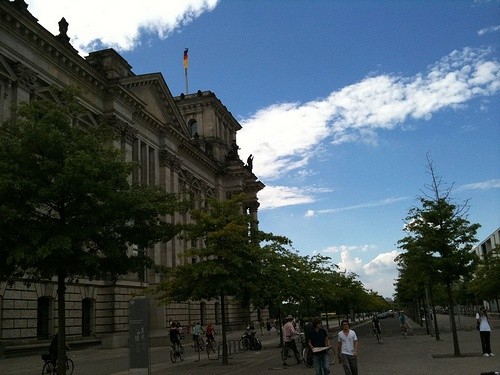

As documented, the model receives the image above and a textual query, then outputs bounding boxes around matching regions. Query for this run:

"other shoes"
[482,352,495,357]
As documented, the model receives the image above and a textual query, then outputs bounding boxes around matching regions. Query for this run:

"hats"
[285,314,293,319]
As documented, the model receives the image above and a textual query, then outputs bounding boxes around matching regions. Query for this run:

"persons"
[48,329,73,368]
[398,311,407,335]
[250,326,256,351]
[246,317,280,336]
[372,314,383,343]
[281,314,303,364]
[335,320,360,375]
[308,320,333,375]
[167,320,217,353]
[475,306,497,358]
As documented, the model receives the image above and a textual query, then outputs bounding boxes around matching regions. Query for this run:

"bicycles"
[193,332,205,351]
[303,343,336,367]
[41,349,74,375]
[205,332,218,353]
[401,323,408,338]
[374,327,382,344]
[169,338,186,363]
[242,325,262,350]
[280,332,307,366]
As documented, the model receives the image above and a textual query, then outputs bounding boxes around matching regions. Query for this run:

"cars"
[320,306,450,324]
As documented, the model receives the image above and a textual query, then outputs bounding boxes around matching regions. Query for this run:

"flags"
[183,50,189,77]
